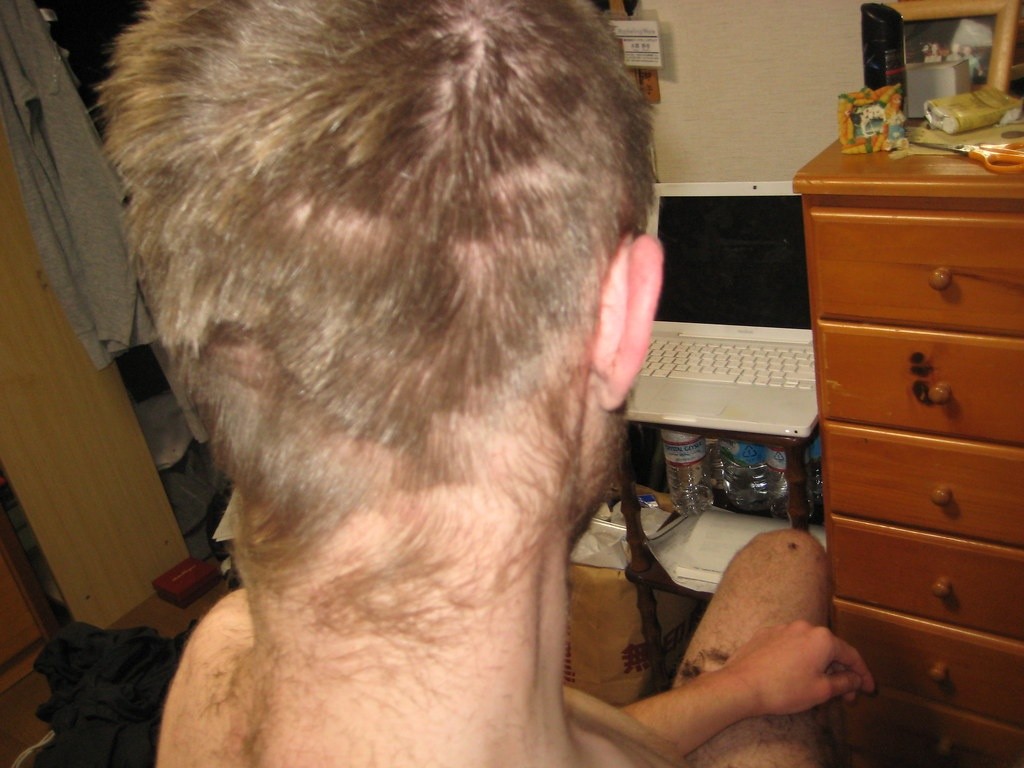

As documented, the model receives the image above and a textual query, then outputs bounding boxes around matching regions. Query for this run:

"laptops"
[622,182,820,438]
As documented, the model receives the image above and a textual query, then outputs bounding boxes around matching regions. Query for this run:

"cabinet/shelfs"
[2,103,191,631]
[615,419,822,695]
[793,119,1024,768]
[0,502,61,695]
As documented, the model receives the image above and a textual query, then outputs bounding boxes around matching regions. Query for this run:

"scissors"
[910,138,1024,176]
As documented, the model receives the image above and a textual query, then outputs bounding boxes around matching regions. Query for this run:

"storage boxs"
[152,555,220,610]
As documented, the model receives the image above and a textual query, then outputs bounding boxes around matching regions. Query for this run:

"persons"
[90,0,877,768]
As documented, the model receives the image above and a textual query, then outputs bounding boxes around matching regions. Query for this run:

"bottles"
[806,434,822,524]
[860,2,906,125]
[662,429,788,518]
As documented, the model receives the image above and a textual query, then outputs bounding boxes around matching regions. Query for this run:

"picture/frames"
[889,1,1021,94]
